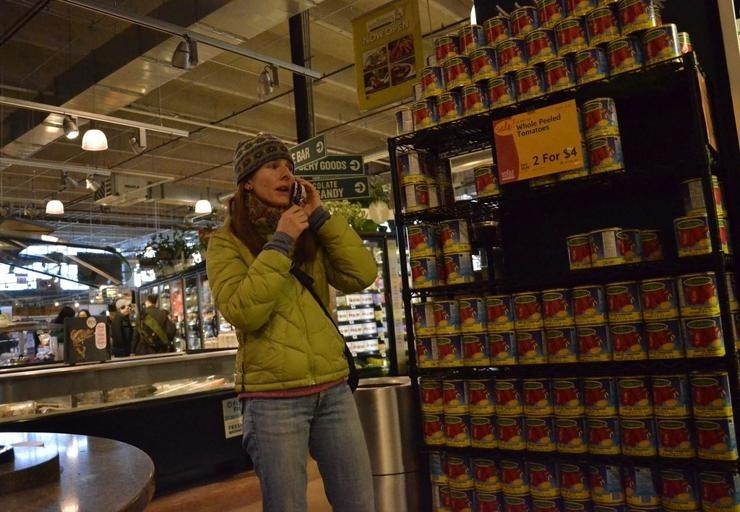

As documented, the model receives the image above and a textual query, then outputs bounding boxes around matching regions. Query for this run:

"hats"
[116,299,132,309]
[233,132,294,186]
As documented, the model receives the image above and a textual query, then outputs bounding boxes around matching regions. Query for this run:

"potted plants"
[150,224,207,281]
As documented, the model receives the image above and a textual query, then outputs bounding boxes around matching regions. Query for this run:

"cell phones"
[290,181,303,202]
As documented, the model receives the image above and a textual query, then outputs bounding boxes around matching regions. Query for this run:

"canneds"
[394,1,740,511]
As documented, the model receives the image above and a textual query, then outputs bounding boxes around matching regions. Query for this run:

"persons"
[206,132,378,512]
[50,294,177,361]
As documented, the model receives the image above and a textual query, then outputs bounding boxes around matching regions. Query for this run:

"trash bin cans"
[355,377,425,512]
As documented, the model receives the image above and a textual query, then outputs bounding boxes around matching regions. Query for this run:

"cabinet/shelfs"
[134,258,236,351]
[387,51,740,511]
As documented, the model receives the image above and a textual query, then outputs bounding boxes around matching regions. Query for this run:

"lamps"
[171,35,281,96]
[61,83,109,152]
[45,198,64,215]
[196,200,212,214]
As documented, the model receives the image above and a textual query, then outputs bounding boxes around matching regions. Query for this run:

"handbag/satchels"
[339,334,359,393]
